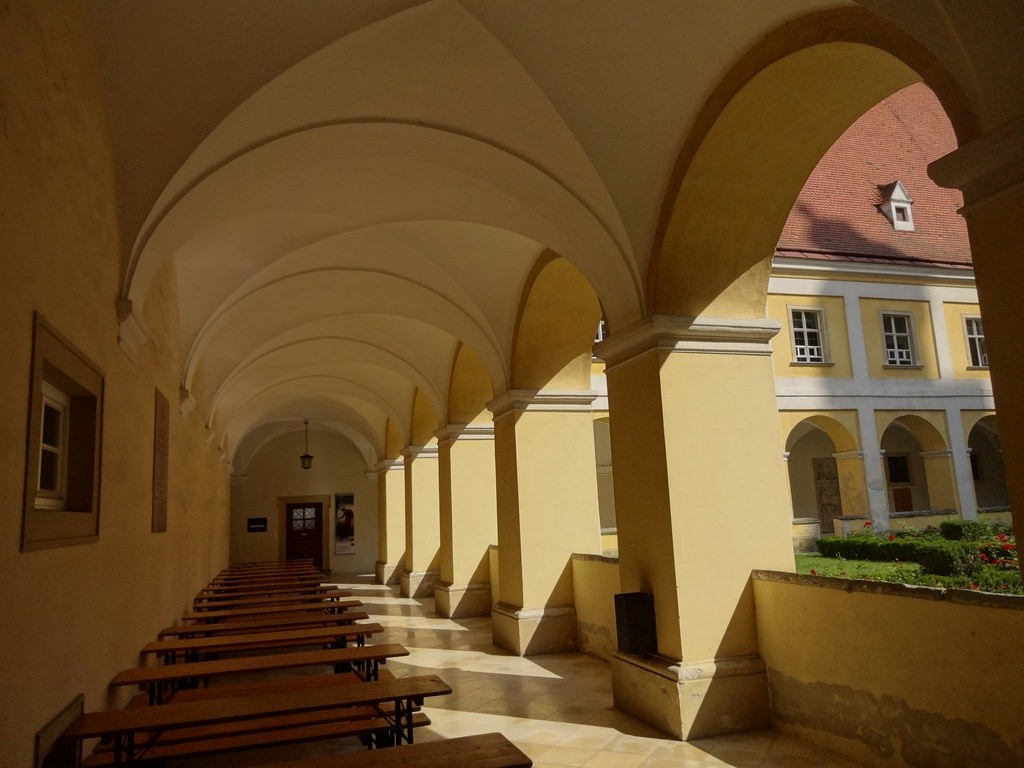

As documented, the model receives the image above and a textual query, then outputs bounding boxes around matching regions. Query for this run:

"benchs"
[64,557,532,768]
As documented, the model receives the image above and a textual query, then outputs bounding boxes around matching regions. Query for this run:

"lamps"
[300,422,313,469]
